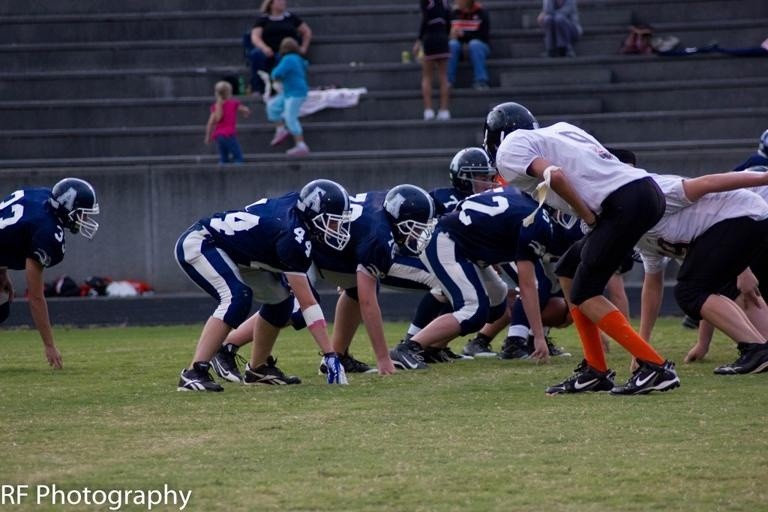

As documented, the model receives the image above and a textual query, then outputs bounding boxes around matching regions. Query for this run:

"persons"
[0,177,100,370]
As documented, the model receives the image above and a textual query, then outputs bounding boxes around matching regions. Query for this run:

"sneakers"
[526,334,572,358]
[498,335,535,361]
[208,339,248,385]
[387,337,429,372]
[421,345,475,366]
[177,360,226,394]
[423,111,451,119]
[243,355,302,386]
[460,338,498,359]
[284,147,310,157]
[681,314,700,330]
[712,335,768,376]
[542,357,617,397]
[268,130,290,147]
[317,347,382,377]
[607,357,682,396]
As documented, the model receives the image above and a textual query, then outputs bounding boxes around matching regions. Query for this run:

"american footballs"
[541,296,568,328]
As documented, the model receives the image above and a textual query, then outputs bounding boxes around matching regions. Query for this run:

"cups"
[402,52,409,64]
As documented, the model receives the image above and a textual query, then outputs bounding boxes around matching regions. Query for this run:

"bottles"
[239,76,245,93]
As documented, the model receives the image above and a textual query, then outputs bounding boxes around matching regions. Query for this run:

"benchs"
[0,0,768,169]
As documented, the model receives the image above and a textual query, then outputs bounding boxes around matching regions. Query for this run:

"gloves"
[316,350,351,386]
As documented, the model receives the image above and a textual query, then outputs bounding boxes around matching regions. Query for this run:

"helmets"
[291,177,355,245]
[447,145,499,200]
[483,101,540,164]
[380,182,438,232]
[45,174,99,235]
[756,128,768,160]
